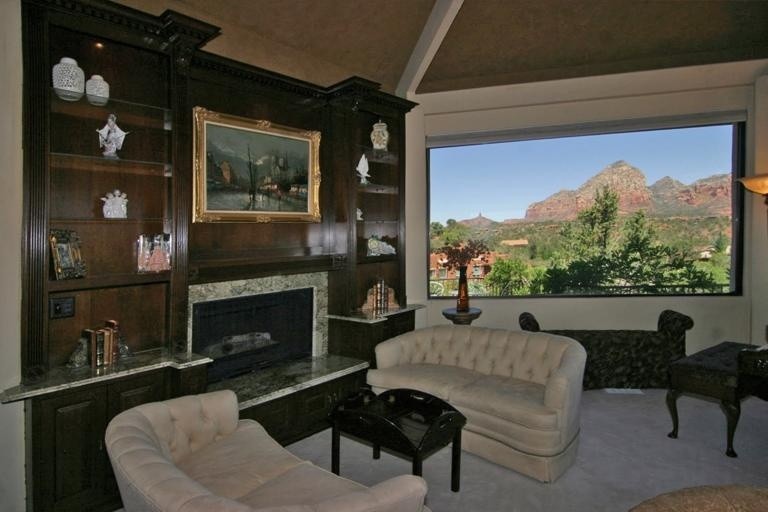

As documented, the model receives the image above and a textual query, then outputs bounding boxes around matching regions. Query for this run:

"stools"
[666,341,761,458]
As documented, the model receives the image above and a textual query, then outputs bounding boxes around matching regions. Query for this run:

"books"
[372,277,389,309]
[82,319,120,368]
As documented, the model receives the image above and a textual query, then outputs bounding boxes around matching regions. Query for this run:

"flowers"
[434,237,490,275]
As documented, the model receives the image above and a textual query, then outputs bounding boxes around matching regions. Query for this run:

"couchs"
[365,326,588,483]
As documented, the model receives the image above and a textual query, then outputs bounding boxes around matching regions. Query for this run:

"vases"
[457,266,469,313]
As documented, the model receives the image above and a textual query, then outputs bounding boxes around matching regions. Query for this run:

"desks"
[737,343,768,401]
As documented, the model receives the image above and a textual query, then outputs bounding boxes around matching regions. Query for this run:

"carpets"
[627,486,768,512]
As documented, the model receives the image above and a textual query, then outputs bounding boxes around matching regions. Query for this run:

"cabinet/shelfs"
[327,311,416,369]
[326,75,419,316]
[104,389,433,512]
[21,0,186,376]
[239,368,369,448]
[31,366,173,512]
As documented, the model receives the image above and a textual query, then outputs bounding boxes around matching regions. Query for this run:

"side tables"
[442,307,482,325]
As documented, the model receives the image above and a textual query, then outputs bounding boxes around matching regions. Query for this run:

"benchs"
[518,309,693,393]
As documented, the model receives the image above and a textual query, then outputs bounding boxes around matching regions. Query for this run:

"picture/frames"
[50,228,88,282]
[192,106,322,224]
